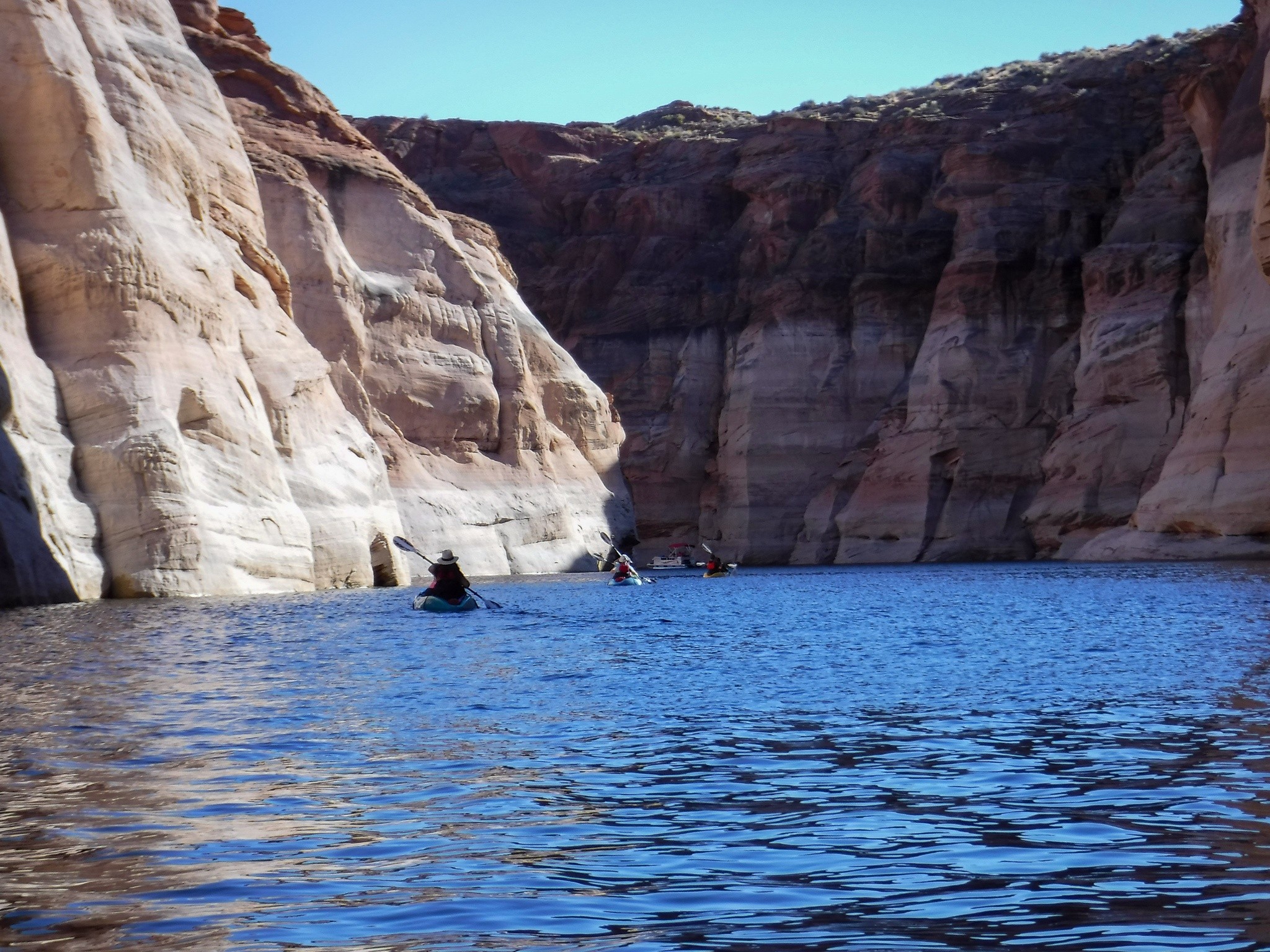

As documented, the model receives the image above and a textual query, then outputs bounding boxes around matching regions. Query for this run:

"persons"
[706,554,726,574]
[428,549,470,605]
[610,554,638,579]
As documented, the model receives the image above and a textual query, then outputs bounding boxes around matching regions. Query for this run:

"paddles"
[701,542,732,575]
[696,561,737,568]
[600,532,646,586]
[592,552,658,584]
[391,536,501,608]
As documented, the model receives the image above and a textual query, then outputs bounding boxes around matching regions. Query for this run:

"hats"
[619,555,628,562]
[437,550,459,565]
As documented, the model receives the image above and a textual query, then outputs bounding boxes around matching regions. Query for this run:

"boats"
[703,568,730,578]
[412,589,480,614]
[646,546,689,570]
[607,573,642,586]
